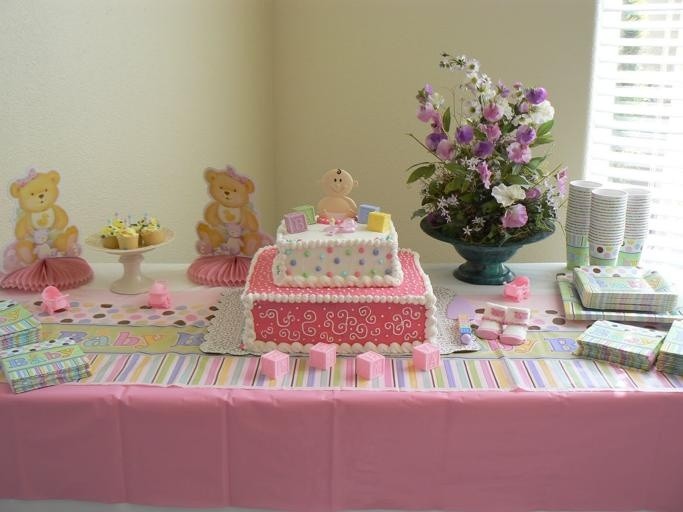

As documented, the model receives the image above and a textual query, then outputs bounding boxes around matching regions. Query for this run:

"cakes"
[244,167,437,351]
[307,341,337,370]
[259,352,289,380]
[100,214,163,250]
[352,352,387,381]
[410,343,442,374]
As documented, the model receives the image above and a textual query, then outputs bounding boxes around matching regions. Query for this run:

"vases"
[416,215,558,289]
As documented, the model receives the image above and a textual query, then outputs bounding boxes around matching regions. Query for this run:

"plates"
[554,263,683,326]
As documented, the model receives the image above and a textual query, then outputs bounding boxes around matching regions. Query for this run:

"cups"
[564,179,651,274]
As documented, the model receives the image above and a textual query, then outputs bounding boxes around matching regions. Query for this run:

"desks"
[0,247,682,508]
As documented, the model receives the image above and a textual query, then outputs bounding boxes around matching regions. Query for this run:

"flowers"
[397,46,576,238]
